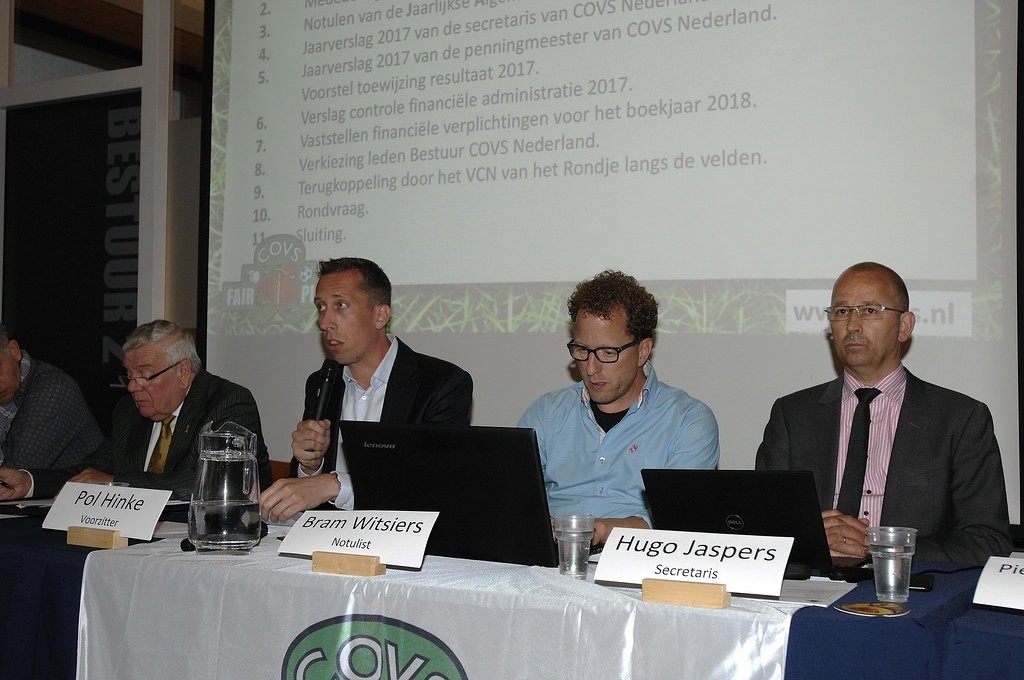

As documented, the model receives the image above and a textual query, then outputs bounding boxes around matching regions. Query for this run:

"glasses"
[825,304,904,321]
[119,359,185,388]
[567,339,640,363]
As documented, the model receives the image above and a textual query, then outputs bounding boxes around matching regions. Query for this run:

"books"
[909,574,934,592]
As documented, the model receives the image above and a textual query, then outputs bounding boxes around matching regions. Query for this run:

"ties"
[147,414,176,474]
[836,388,882,521]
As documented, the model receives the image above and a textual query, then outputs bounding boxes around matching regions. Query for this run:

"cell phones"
[909,575,935,592]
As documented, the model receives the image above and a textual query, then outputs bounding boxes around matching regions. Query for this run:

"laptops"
[340,421,606,567]
[641,468,873,577]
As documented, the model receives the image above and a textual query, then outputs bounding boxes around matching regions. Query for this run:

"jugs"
[187,420,262,555]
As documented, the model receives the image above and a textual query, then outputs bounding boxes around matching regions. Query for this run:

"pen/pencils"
[0,480,15,490]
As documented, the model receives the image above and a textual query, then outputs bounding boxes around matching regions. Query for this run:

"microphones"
[313,359,340,422]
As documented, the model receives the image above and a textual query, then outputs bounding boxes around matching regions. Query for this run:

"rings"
[844,537,846,543]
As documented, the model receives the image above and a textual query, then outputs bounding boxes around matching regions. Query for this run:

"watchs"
[335,473,341,486]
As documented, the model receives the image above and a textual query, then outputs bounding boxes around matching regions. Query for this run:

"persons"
[755,262,1012,574]
[260,258,474,522]
[516,272,721,545]
[0,319,273,500]
[0,324,105,469]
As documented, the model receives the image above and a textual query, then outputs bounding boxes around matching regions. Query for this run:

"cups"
[94,482,129,487]
[866,526,918,603]
[554,514,597,580]
